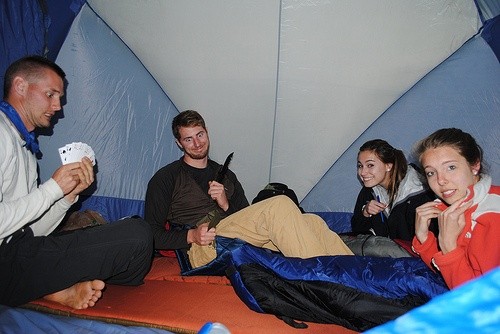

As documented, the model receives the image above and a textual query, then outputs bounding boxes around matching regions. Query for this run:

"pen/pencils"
[377,195,385,224]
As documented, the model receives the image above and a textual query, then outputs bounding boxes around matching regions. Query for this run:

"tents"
[0,0,500,334]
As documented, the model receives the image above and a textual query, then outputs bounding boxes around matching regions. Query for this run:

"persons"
[144,110,354,277]
[0,54,155,309]
[410,126,500,289]
[351,138,450,257]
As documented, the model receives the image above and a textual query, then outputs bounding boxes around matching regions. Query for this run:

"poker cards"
[58,141,96,167]
